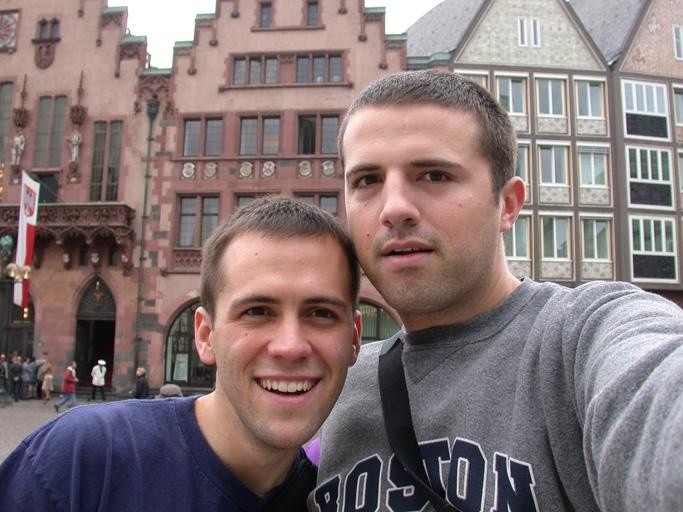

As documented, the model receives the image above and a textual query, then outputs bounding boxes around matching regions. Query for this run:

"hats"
[96,359,106,365]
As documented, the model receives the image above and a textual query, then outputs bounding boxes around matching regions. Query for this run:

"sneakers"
[54,403,59,413]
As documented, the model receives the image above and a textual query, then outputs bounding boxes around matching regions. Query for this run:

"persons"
[156,385,182,398]
[53,360,80,413]
[88,359,109,403]
[0,349,54,405]
[133,365,151,399]
[0,193,365,512]
[300,65,682,512]
